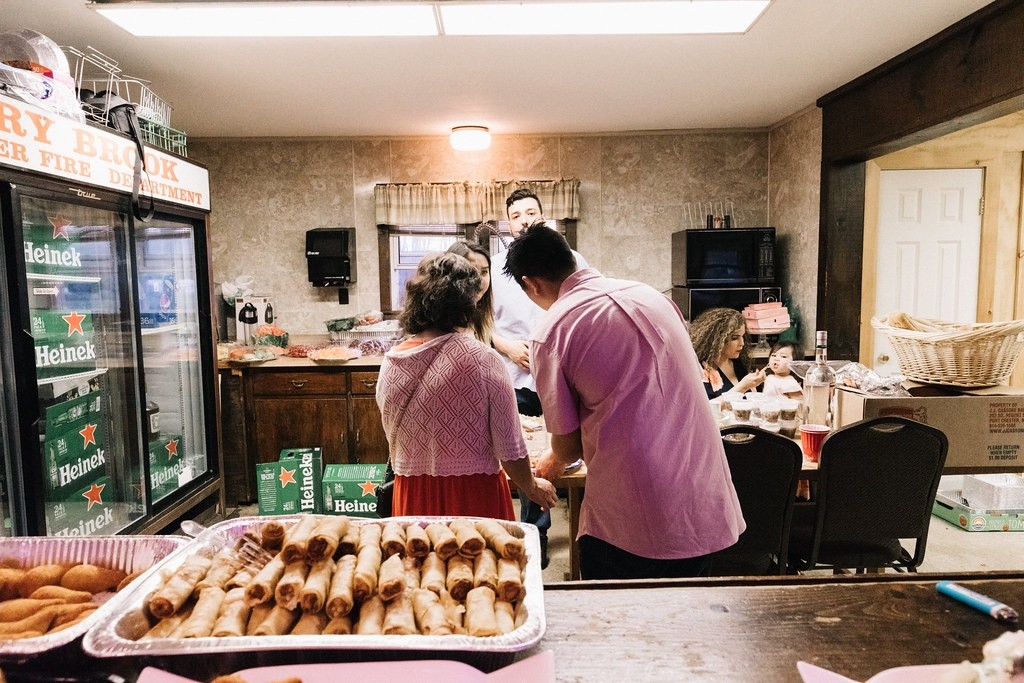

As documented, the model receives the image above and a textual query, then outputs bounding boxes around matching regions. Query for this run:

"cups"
[799,424,831,463]
[710,391,798,440]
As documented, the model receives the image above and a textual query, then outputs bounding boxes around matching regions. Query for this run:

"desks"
[508,420,834,581]
[511,569,1023,682]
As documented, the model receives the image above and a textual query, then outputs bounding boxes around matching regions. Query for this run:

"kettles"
[239,302,259,347]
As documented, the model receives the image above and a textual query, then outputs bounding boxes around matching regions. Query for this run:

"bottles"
[800,330,836,431]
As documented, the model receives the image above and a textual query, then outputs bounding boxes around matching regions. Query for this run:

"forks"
[180,520,275,575]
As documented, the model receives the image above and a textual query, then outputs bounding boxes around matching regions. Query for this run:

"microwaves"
[671,227,777,286]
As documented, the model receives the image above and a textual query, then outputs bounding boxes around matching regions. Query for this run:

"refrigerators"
[0,119,225,539]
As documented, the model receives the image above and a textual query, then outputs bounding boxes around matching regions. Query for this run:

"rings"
[549,501,554,505]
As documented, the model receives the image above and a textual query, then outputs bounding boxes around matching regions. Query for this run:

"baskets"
[872,311,1023,386]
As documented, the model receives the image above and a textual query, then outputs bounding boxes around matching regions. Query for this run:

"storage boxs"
[255,449,388,517]
[827,383,1023,474]
[233,296,274,346]
[21,223,184,537]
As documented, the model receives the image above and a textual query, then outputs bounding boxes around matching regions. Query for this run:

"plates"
[746,328,786,353]
[529,458,583,474]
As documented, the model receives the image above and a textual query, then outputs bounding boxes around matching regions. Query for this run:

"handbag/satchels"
[75,88,155,222]
[376,480,393,517]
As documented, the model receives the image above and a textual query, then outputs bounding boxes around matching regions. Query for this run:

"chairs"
[783,415,950,574]
[715,423,804,576]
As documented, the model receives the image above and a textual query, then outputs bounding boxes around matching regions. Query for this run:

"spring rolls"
[136,515,525,638]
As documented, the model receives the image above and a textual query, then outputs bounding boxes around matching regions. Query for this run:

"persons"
[501,217,749,581]
[445,240,494,352]
[686,308,766,408]
[375,250,558,520]
[490,189,592,418]
[762,340,804,400]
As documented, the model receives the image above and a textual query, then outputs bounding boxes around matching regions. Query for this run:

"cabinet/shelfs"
[244,366,391,502]
